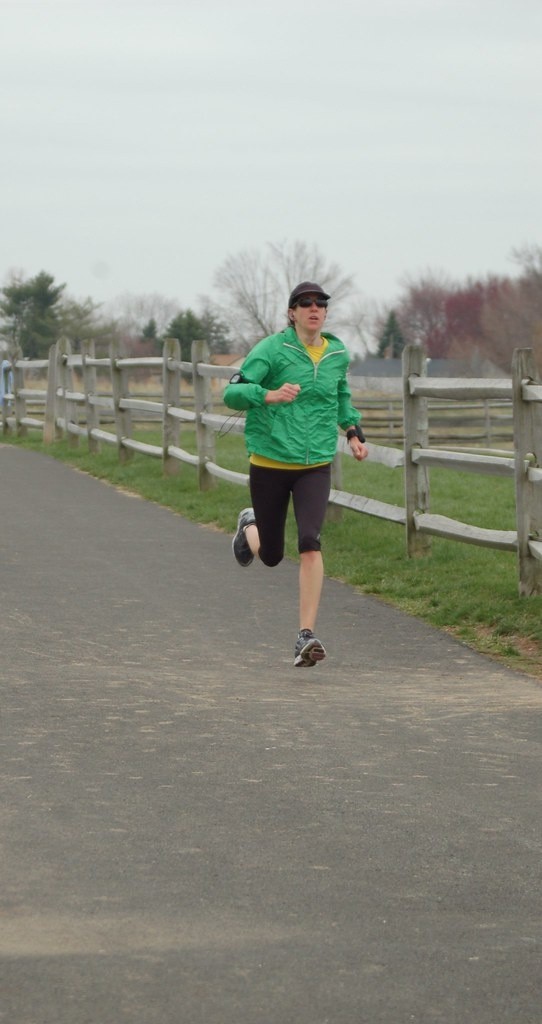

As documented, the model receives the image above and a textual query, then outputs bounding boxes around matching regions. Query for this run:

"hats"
[289,282,331,308]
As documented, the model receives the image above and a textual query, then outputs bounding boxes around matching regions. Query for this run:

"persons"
[223,282,368,667]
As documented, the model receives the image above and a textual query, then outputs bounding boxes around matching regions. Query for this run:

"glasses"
[292,298,328,309]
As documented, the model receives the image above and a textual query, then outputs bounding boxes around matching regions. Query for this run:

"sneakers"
[232,508,258,568]
[294,629,327,667]
